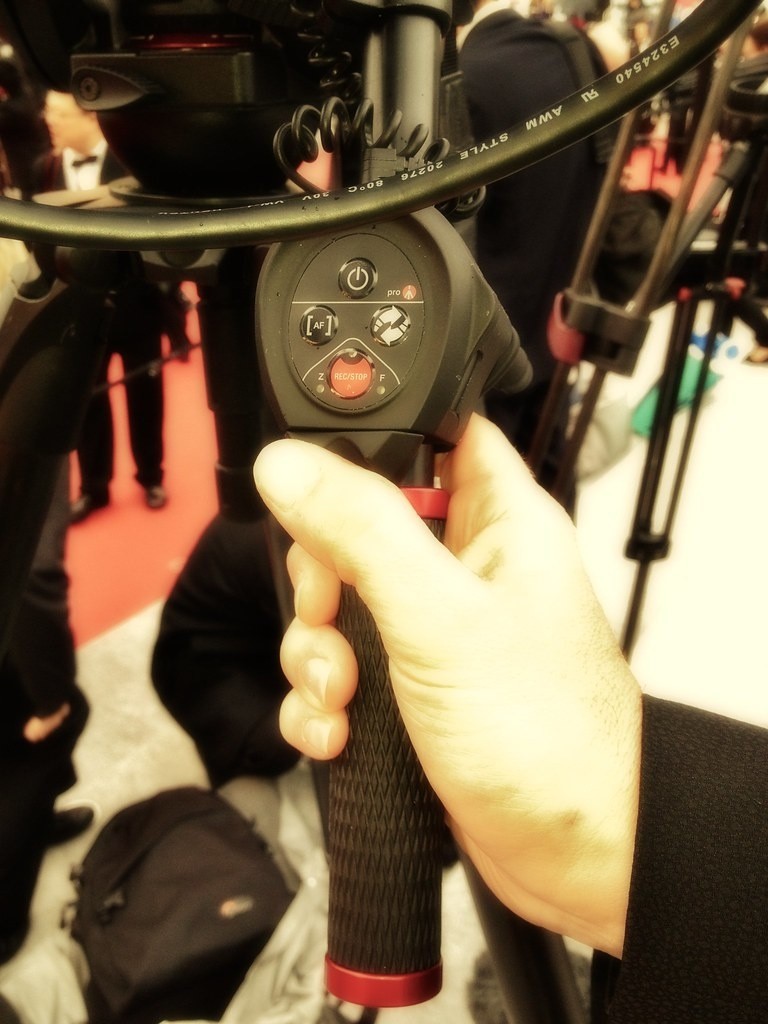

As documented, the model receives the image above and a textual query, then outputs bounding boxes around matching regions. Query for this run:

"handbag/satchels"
[68,786,295,1024]
[594,185,674,301]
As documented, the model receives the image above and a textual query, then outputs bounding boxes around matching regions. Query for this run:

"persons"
[0,0,766,1021]
[251,411,768,1024]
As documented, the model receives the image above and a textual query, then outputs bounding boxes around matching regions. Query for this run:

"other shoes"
[47,806,93,845]
[68,487,108,521]
[146,483,167,508]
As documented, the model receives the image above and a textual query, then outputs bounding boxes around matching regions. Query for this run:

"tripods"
[531,0,767,665]
